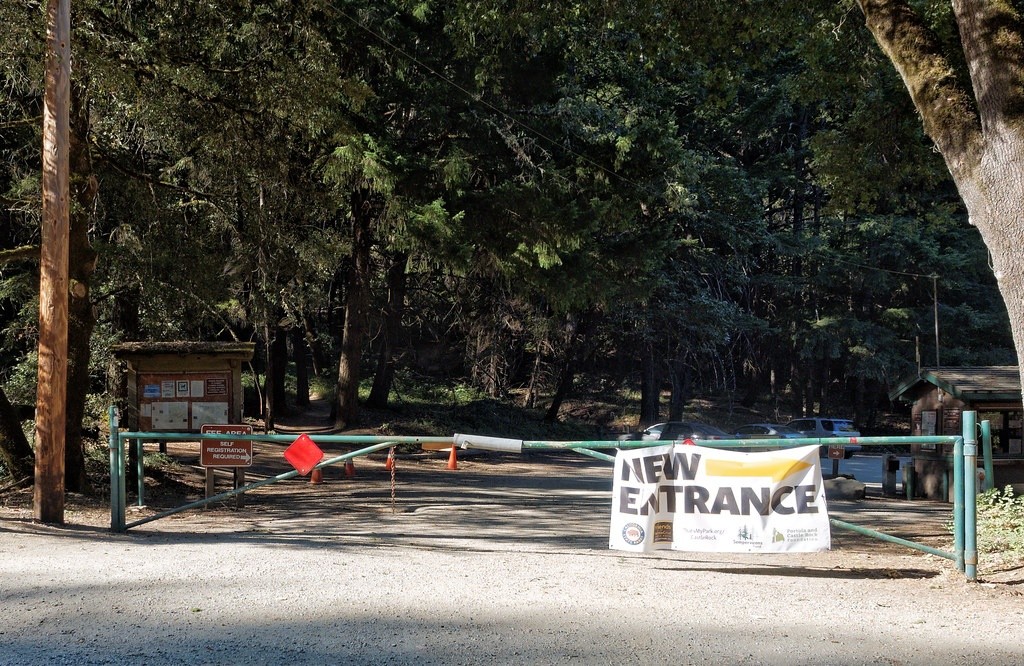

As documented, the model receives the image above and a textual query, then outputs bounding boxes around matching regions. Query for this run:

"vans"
[785,417,862,459]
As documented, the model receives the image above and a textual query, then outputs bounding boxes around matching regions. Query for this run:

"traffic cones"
[306,461,328,486]
[444,444,460,471]
[383,447,399,471]
[342,447,356,479]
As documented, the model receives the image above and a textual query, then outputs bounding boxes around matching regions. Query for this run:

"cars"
[617,421,751,454]
[728,424,808,453]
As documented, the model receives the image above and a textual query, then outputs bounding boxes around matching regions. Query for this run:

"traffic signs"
[199,425,253,468]
